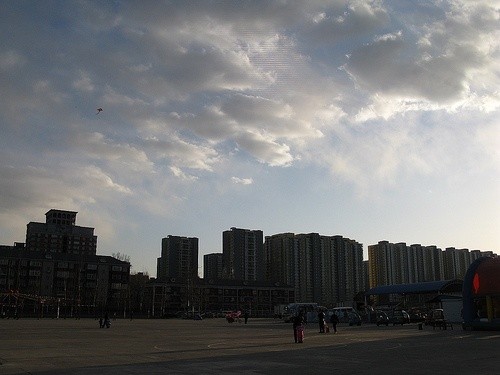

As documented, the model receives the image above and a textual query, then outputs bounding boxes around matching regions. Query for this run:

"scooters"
[349,313,362,326]
[377,310,410,326]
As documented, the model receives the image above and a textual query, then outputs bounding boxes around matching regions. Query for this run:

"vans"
[334,307,355,312]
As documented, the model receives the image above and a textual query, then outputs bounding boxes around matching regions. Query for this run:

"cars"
[193,311,233,320]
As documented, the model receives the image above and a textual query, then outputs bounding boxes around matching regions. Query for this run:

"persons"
[99,318,111,328]
[293,309,337,343]
[244,311,248,324]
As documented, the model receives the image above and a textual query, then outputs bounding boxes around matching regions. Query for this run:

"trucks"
[429,299,463,326]
[283,303,328,321]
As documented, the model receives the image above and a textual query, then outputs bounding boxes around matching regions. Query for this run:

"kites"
[96,108,103,115]
